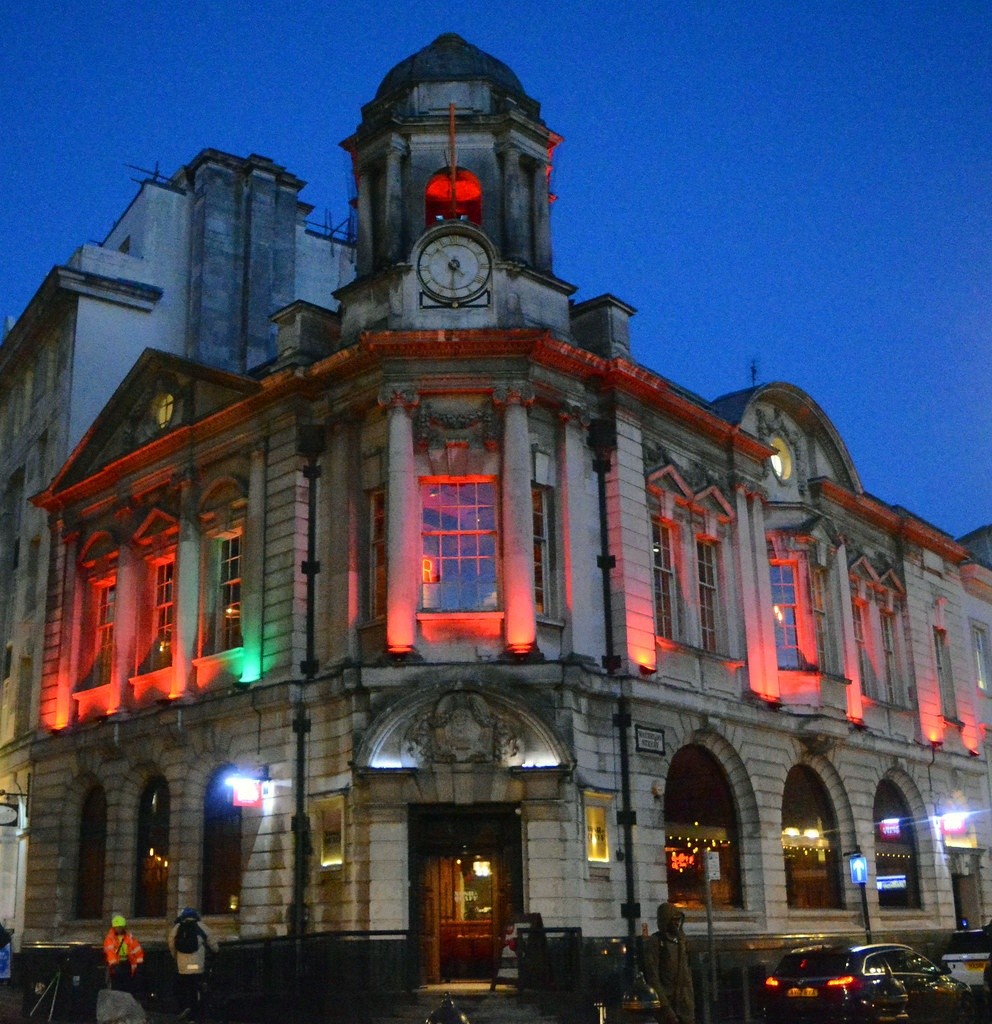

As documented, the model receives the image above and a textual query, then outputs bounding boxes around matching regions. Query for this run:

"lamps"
[233,681,250,692]
[153,697,173,709]
[47,727,65,737]
[929,739,944,750]
[514,651,530,665]
[969,749,981,759]
[93,715,108,725]
[854,723,870,733]
[639,664,657,677]
[767,702,785,710]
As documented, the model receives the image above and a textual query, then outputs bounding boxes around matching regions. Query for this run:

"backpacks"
[176,919,199,953]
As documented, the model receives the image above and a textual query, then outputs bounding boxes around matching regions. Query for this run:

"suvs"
[940,929,991,1003]
[762,942,978,1024]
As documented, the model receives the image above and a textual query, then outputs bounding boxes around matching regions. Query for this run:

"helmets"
[183,907,197,917]
[111,915,126,927]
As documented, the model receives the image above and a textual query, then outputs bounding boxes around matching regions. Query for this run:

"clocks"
[417,235,494,302]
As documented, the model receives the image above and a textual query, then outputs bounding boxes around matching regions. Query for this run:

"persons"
[103,915,144,999]
[168,906,218,1024]
[641,903,696,1024]
